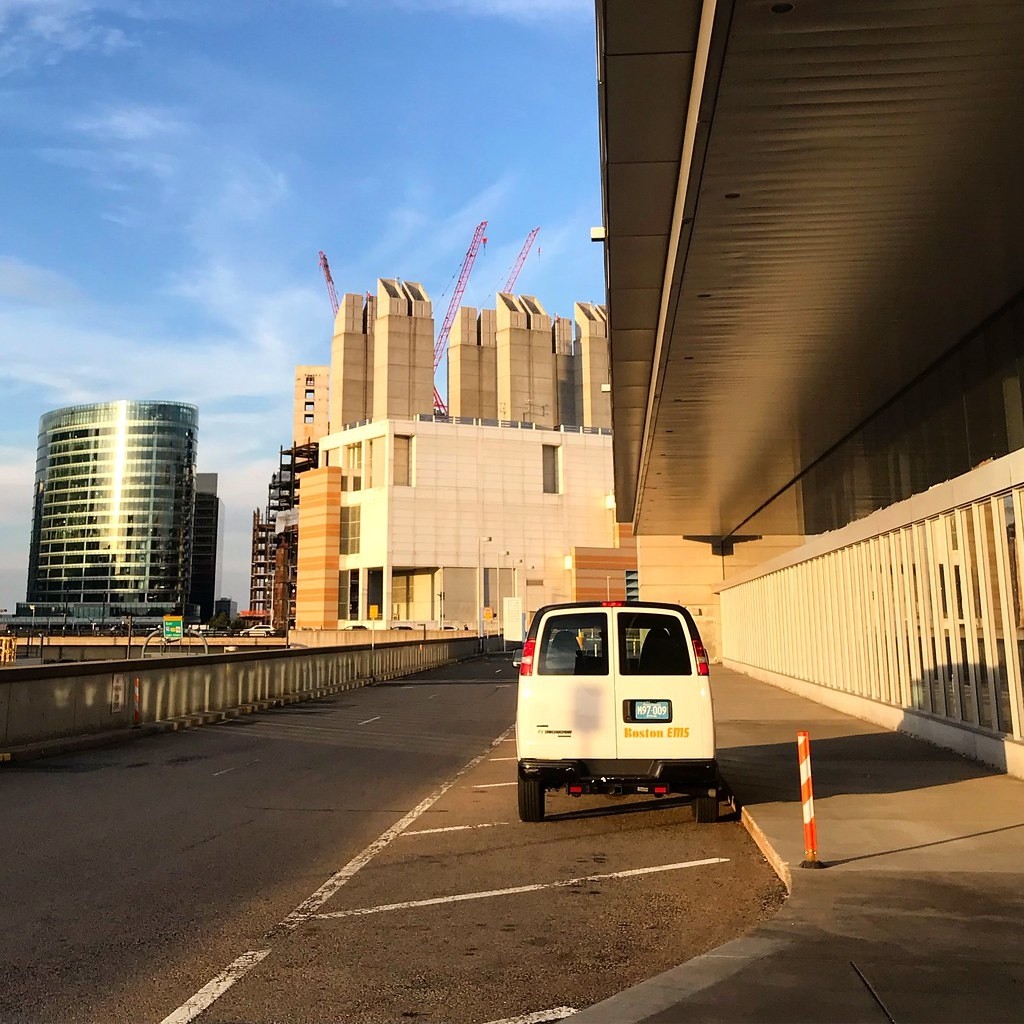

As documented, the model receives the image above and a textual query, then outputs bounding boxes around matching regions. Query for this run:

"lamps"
[564,555,573,572]
[590,226,605,242]
[605,494,615,510]
[601,382,610,394]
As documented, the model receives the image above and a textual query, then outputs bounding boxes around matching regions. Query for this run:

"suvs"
[239,624,277,637]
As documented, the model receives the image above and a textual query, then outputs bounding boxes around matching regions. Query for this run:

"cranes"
[318,220,542,411]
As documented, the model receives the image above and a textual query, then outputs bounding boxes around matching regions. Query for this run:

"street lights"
[512,559,522,596]
[268,571,275,627]
[28,604,35,636]
[494,550,509,653]
[523,565,534,636]
[476,536,492,653]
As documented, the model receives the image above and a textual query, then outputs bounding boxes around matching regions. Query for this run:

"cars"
[434,625,459,631]
[343,626,367,630]
[198,625,234,637]
[389,626,412,631]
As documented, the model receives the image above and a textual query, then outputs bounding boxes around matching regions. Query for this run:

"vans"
[511,599,722,824]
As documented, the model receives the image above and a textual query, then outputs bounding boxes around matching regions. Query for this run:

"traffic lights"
[440,591,445,601]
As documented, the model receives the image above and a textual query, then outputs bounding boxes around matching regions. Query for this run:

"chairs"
[636,626,682,676]
[548,631,583,669]
